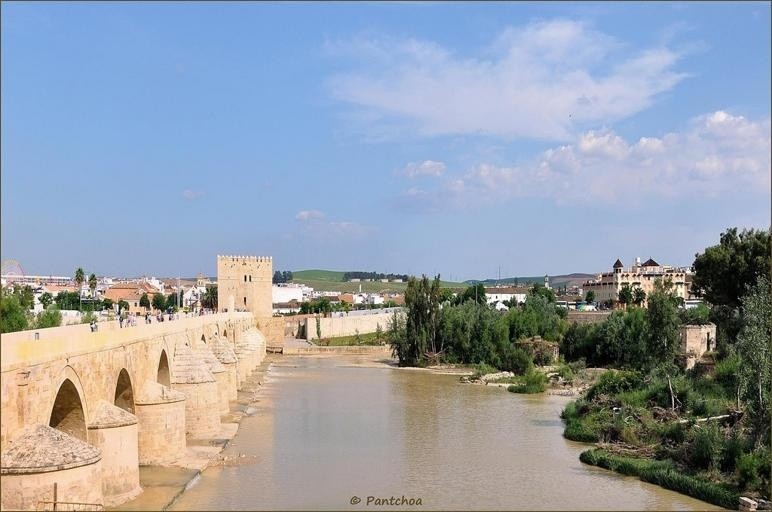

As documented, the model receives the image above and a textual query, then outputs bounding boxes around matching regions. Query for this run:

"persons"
[90,306,248,332]
